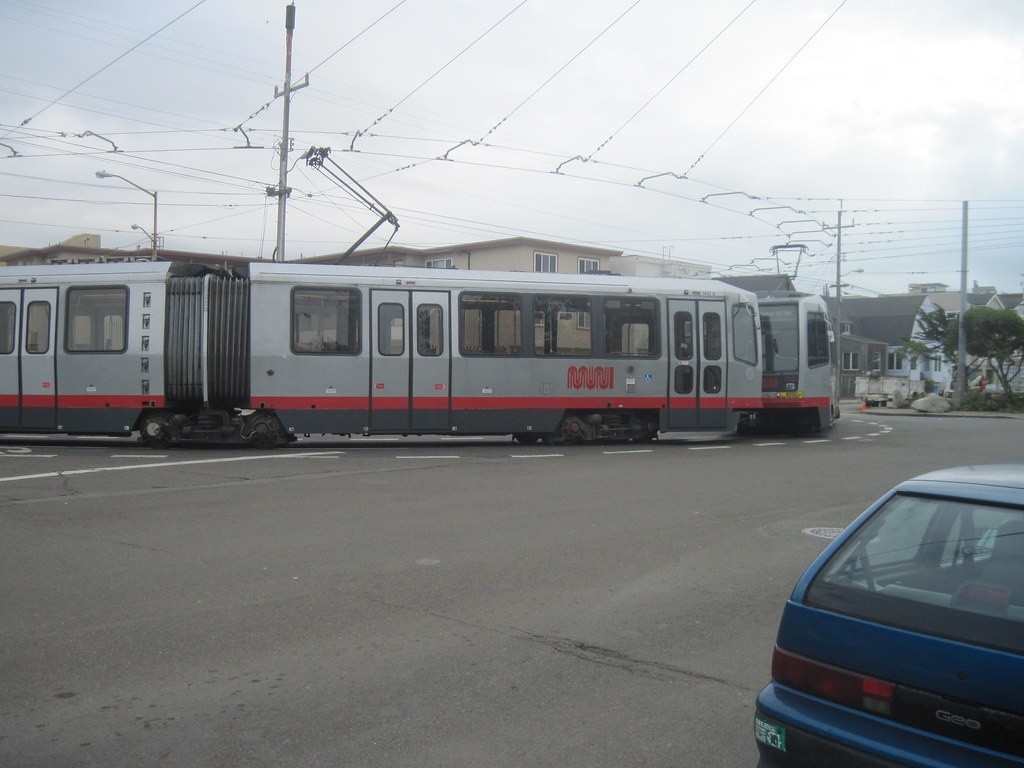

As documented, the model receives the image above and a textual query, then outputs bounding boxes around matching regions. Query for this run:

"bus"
[0,252,766,449]
[738,291,840,436]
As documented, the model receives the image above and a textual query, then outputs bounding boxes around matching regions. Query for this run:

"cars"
[751,464,1024,768]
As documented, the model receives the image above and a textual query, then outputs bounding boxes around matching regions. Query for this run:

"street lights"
[834,268,864,400]
[95,169,159,262]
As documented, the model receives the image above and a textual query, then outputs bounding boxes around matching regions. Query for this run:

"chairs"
[984,519,1024,605]
